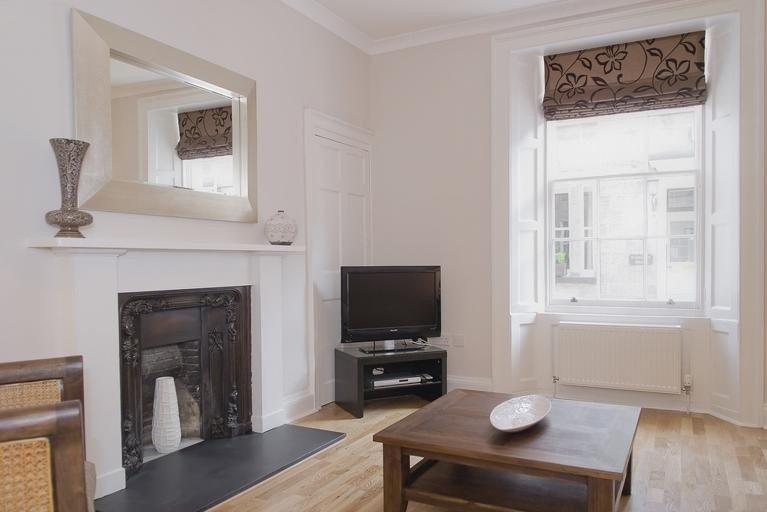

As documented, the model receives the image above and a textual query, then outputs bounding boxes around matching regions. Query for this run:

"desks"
[372,384,642,511]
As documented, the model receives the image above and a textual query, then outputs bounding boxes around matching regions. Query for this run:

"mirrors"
[67,7,260,225]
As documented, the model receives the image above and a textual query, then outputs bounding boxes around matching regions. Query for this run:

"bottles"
[263,210,299,245]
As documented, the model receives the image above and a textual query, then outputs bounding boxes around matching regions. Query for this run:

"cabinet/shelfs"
[332,344,448,417]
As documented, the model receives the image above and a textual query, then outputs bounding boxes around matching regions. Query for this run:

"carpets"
[95,422,347,511]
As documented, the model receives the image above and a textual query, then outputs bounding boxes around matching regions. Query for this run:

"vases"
[46,138,92,238]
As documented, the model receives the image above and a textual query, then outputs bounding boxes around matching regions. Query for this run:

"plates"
[489,395,553,433]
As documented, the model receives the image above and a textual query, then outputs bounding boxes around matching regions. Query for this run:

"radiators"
[547,320,689,398]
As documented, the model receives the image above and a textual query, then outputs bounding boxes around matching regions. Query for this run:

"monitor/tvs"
[341,266,441,353]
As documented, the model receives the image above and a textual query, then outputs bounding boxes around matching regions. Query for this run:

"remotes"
[422,374,433,380]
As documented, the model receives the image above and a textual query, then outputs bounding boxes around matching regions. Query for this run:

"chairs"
[1,355,97,510]
